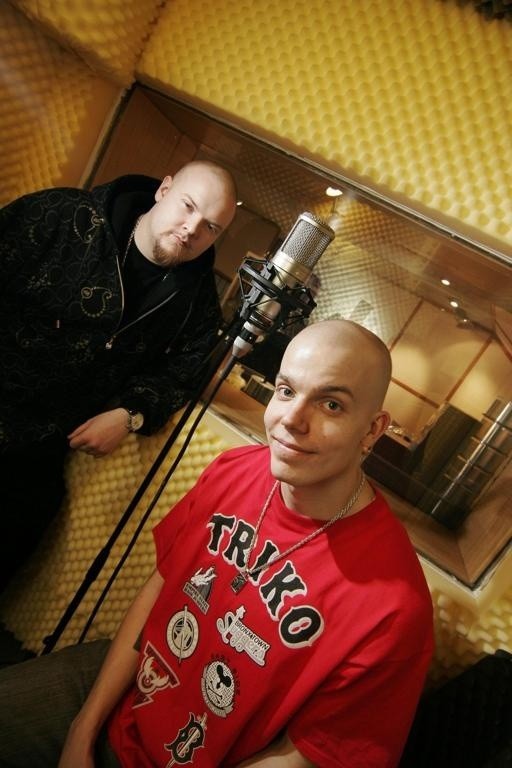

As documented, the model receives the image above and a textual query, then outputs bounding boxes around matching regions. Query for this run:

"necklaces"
[226,466,366,596]
[122,211,174,284]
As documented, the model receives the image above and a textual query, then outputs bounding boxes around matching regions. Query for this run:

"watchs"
[123,404,145,433]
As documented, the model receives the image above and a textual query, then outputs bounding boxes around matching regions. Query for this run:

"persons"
[0,320,437,768]
[1,158,241,606]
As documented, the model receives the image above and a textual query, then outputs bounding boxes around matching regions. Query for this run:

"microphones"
[232,210,335,358]
